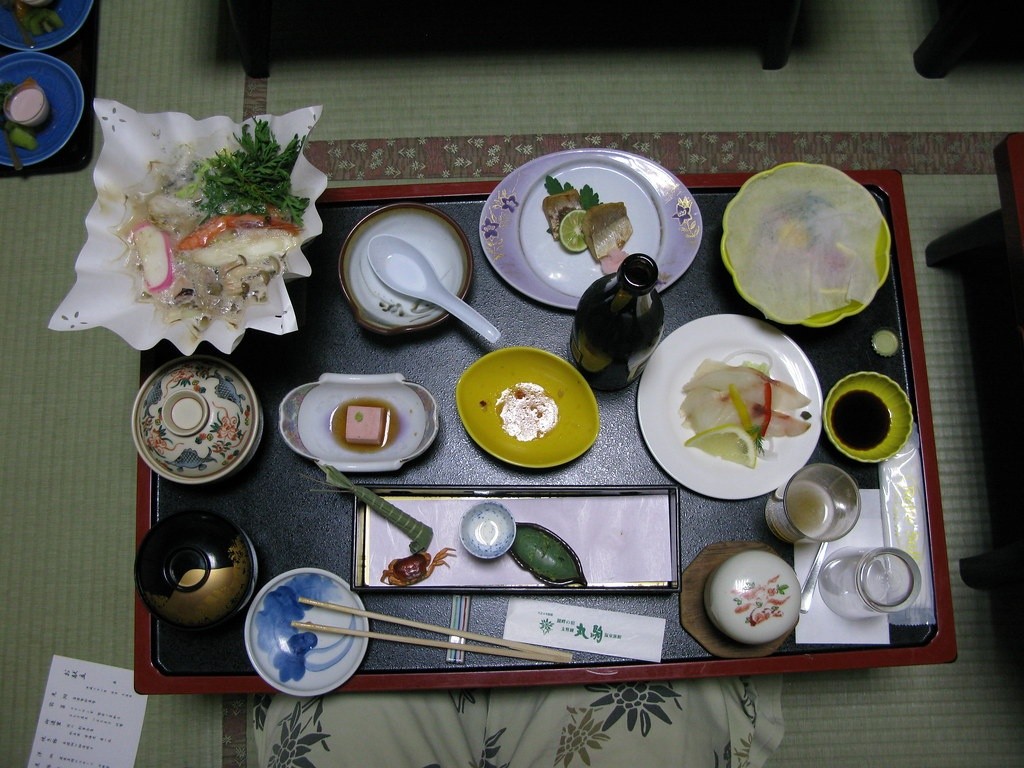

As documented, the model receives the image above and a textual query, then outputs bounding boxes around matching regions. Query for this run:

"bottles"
[572,255,668,393]
[134,508,259,632]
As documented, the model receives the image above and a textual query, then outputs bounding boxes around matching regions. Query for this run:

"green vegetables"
[544,175,603,234]
[747,426,765,456]
[179,115,311,228]
[0,81,37,136]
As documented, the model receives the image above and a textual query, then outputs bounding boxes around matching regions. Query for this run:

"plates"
[479,147,705,310]
[638,313,825,499]
[823,371,915,463]
[0,0,93,51]
[723,162,891,327]
[794,489,890,645]
[338,202,473,335]
[0,52,85,170]
[245,567,369,697]
[277,375,441,475]
[460,498,516,560]
[505,519,587,588]
[452,346,601,469]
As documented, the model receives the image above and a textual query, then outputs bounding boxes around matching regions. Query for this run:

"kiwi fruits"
[23,8,64,37]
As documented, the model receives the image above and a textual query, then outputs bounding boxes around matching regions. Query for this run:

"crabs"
[380,544,457,586]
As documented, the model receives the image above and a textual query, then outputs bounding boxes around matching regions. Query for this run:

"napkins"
[792,489,891,646]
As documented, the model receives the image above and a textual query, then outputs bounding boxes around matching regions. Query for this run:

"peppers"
[728,383,772,437]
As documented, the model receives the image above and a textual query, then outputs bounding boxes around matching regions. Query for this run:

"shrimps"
[178,214,301,250]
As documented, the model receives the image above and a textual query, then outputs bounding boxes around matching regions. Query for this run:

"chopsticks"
[291,596,573,665]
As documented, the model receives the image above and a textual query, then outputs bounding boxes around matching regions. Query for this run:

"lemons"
[559,210,590,253]
[684,424,757,469]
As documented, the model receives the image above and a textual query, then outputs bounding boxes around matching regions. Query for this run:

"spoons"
[367,234,501,343]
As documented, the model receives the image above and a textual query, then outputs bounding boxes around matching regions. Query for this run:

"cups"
[765,465,862,544]
[816,547,921,621]
[129,354,264,488]
[3,77,51,131]
[704,550,802,646]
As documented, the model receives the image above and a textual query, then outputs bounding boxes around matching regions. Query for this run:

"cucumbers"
[9,128,38,151]
